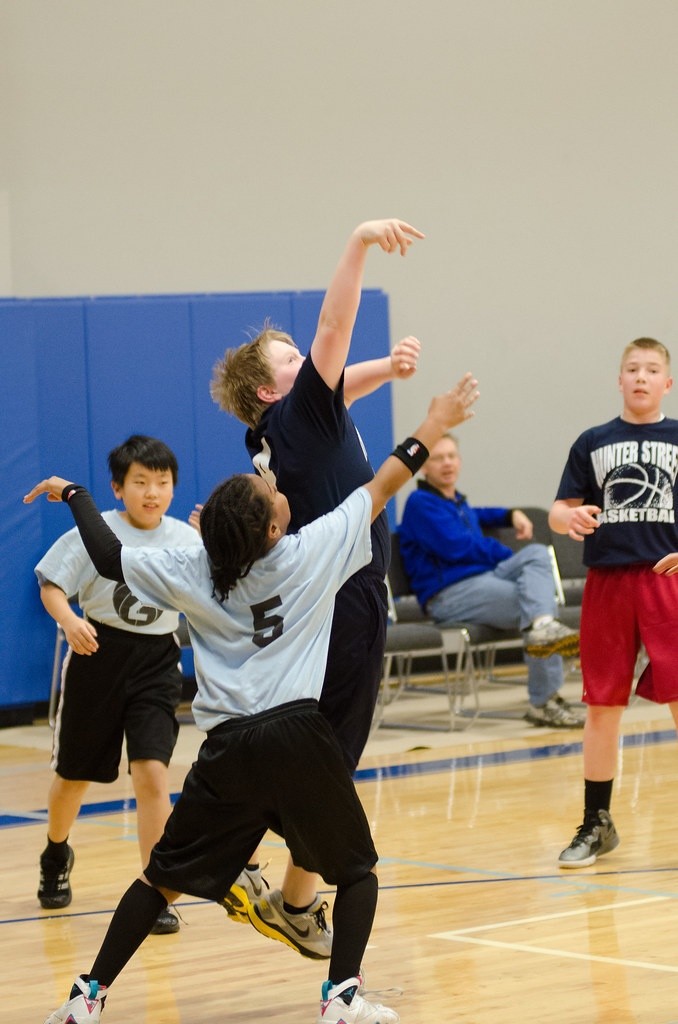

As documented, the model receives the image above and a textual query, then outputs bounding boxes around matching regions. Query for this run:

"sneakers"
[247,888,333,962]
[522,694,586,729]
[149,907,182,935]
[215,863,271,924]
[317,975,401,1024]
[524,620,580,660]
[35,843,75,909]
[41,973,108,1024]
[557,808,620,869]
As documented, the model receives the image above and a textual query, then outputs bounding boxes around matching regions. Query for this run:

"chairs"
[369,509,589,726]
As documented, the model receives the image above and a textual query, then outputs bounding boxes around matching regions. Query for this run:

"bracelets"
[389,436,430,477]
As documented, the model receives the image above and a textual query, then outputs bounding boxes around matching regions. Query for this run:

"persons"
[398,434,585,729]
[33,436,203,935]
[24,374,480,1024]
[210,217,427,959]
[547,338,678,866]
